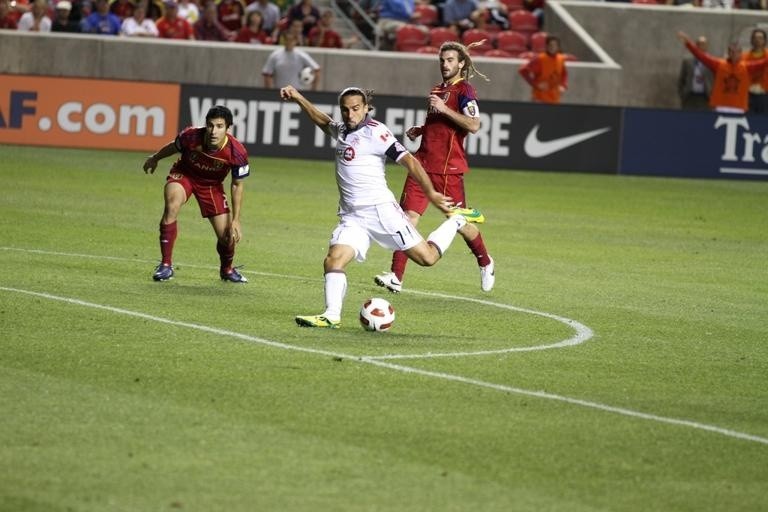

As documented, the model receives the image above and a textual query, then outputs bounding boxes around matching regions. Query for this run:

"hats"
[55,0,72,11]
[162,0,179,9]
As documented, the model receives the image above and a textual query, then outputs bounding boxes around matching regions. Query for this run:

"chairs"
[391,1,578,62]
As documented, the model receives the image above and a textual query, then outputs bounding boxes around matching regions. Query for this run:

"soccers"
[358,298,396,332]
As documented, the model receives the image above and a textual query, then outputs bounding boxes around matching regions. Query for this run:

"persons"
[677,29,768,113]
[277,85,487,331]
[260,31,324,94]
[741,30,768,113]
[142,104,252,286]
[677,35,718,112]
[516,36,570,105]
[372,40,498,295]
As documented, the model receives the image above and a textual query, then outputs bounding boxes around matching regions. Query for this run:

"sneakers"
[374,271,403,293]
[295,314,343,330]
[478,253,495,292]
[153,264,174,281]
[219,267,248,283]
[448,206,485,224]
